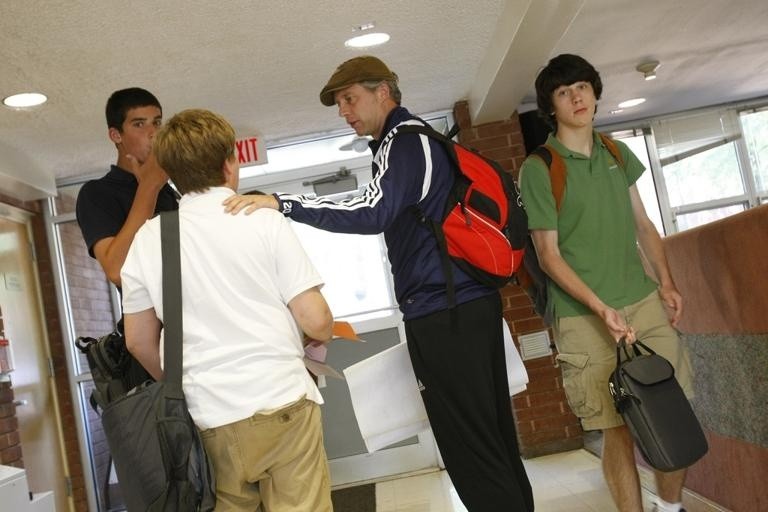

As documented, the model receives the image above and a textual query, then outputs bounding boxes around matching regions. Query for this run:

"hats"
[320,56,397,106]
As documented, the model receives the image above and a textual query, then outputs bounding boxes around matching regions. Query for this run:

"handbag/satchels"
[100,378,216,511]
[608,352,709,472]
[84,332,148,409]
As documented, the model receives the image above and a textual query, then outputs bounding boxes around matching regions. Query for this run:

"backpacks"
[512,132,624,320]
[374,115,528,291]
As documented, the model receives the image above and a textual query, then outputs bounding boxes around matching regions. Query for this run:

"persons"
[518,53,696,512]
[120,108,337,512]
[77,87,181,297]
[223,56,536,512]
